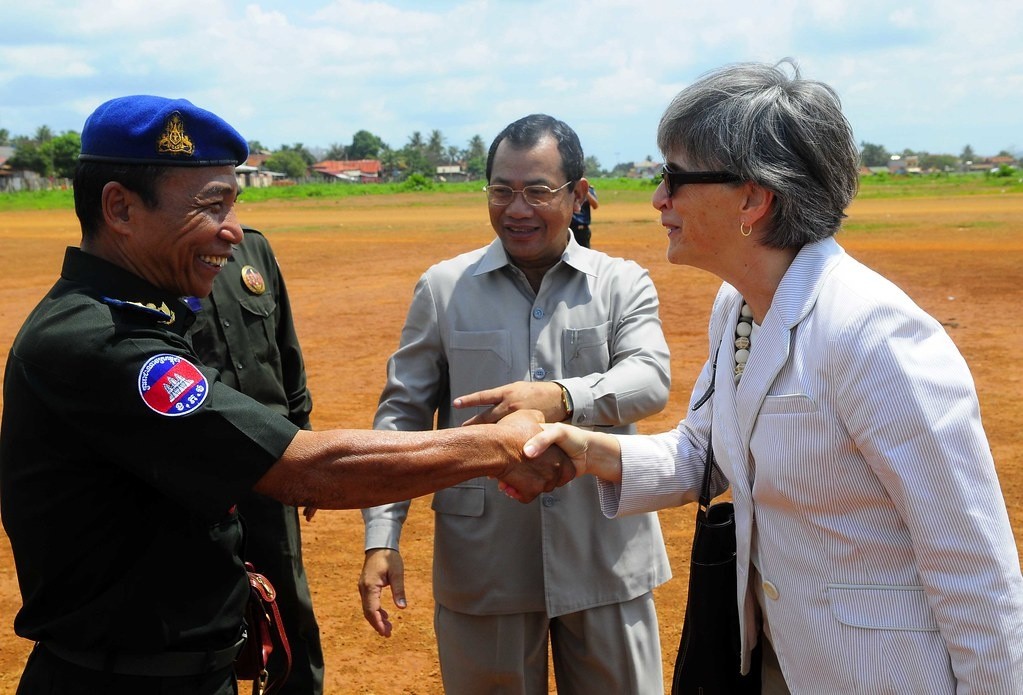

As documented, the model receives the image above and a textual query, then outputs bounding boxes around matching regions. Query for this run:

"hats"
[78,95,250,166]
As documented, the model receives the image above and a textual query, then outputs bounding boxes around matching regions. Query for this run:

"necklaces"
[733,301,756,386]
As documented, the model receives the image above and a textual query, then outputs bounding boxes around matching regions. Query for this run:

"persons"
[570,187,599,249]
[487,62,1022,695]
[358,114,671,695]
[0,95,579,695]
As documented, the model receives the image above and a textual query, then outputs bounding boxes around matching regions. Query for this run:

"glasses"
[662,164,744,197]
[483,181,572,207]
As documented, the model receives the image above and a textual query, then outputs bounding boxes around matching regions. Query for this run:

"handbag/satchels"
[671,337,762,695]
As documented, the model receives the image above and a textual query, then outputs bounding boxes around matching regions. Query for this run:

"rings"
[547,452,574,477]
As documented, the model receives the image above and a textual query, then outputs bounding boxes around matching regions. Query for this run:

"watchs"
[559,384,574,420]
